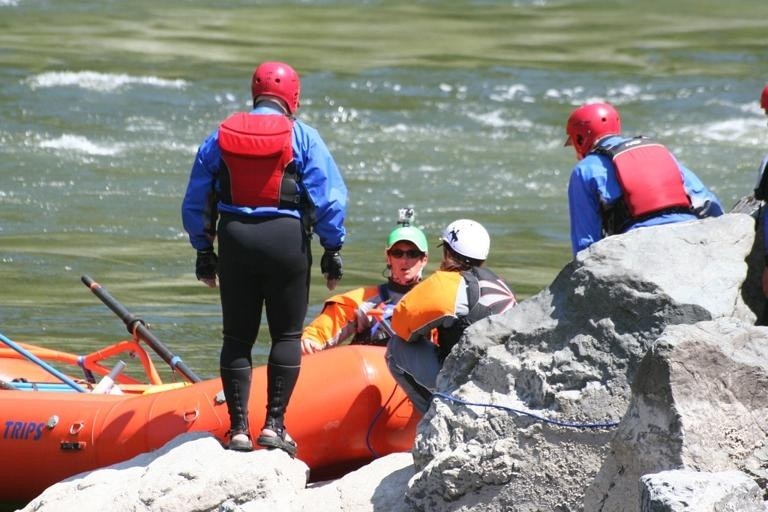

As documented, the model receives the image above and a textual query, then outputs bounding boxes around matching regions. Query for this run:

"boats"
[0,345,423,503]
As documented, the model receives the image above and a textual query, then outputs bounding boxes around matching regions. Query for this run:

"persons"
[300,208,438,355]
[385,219,519,417]
[754,84,768,296]
[564,103,726,262]
[180,61,349,457]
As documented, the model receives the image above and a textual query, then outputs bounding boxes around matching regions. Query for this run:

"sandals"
[258,424,298,454]
[228,430,253,450]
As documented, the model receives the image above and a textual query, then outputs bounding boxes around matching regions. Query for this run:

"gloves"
[195,248,219,279]
[321,251,343,279]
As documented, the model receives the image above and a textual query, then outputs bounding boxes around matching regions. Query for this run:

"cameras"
[398,208,415,223]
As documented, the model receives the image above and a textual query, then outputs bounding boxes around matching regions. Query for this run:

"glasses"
[390,249,422,260]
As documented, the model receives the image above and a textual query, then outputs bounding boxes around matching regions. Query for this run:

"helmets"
[252,61,299,114]
[566,103,622,154]
[442,218,490,260]
[386,226,428,253]
